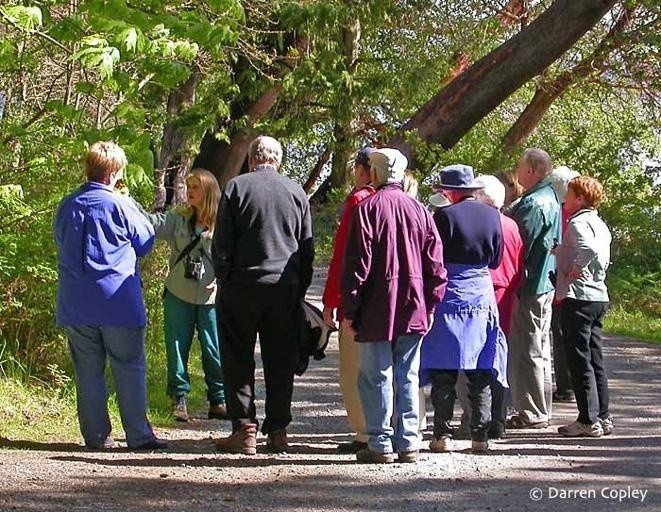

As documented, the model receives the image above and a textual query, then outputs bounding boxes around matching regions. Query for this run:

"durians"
[184,256,206,280]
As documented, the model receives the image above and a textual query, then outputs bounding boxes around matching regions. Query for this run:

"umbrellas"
[128,438,168,450]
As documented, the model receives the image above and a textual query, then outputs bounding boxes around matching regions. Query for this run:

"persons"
[211,135,317,455]
[114,167,230,420]
[55,139,169,450]
[319,146,614,463]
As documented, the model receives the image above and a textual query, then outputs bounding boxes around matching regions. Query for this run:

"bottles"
[356,146,370,165]
[434,164,486,190]
[368,147,408,176]
[429,191,450,207]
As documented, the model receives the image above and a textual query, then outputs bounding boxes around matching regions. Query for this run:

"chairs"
[215,424,258,455]
[170,395,189,421]
[487,429,506,439]
[208,402,232,419]
[398,449,417,462]
[558,418,604,437]
[506,413,548,429]
[471,440,489,452]
[266,432,288,451]
[337,440,367,453]
[596,415,616,434]
[550,391,576,402]
[84,437,114,452]
[357,447,395,464]
[429,437,451,452]
